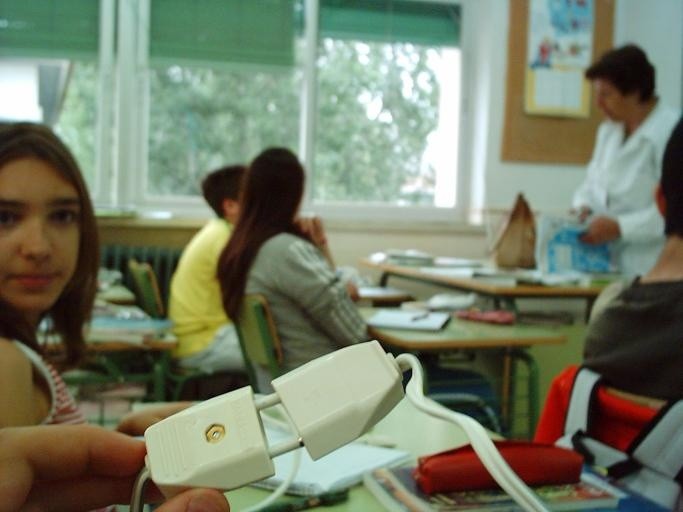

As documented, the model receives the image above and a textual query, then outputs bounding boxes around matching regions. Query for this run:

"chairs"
[226,292,292,395]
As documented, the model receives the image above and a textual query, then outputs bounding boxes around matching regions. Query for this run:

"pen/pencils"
[261,492,348,511]
[412,313,430,321]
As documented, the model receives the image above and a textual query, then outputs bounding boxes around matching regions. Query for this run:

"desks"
[127,398,663,512]
[358,303,569,442]
[355,251,608,336]
[37,302,178,410]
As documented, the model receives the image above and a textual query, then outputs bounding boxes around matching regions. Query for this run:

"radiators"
[98,242,184,320]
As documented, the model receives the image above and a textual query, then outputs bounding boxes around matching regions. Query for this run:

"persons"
[166,163,246,379]
[214,147,488,409]
[0,120,194,425]
[585,110,682,398]
[570,43,676,279]
[0,427,231,511]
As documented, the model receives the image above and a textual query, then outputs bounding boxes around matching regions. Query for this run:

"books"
[90,309,171,347]
[246,434,419,498]
[384,246,548,287]
[363,466,619,511]
[365,307,453,332]
